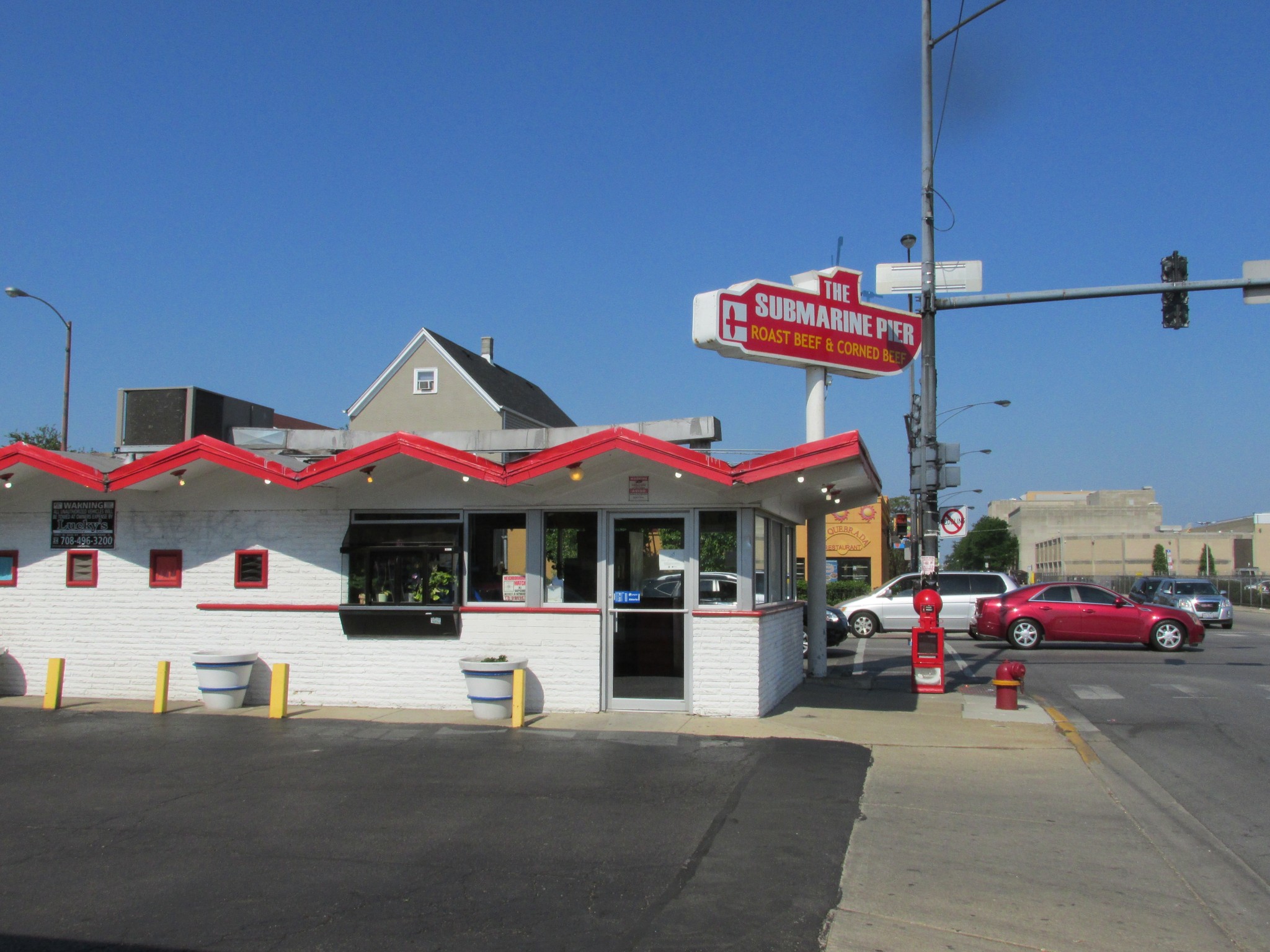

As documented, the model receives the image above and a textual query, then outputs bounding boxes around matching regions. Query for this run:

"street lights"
[5,287,72,454]
[907,358,1012,571]
[901,233,920,421]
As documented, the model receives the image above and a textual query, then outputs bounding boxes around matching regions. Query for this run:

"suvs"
[1129,575,1177,604]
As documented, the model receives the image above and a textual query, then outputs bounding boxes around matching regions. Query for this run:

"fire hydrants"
[991,658,1028,710]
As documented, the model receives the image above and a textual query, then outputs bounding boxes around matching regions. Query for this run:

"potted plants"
[348,564,392,602]
[460,654,529,720]
[403,570,452,602]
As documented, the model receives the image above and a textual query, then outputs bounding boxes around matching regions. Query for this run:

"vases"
[191,649,259,709]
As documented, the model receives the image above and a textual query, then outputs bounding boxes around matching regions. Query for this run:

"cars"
[833,570,1022,640]
[1152,579,1233,629]
[973,581,1205,656]
[1246,580,1270,595]
[644,572,850,659]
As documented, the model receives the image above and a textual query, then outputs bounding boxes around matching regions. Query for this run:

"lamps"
[463,474,469,482]
[826,484,834,500]
[0,473,15,488]
[360,465,376,483]
[170,469,187,486]
[797,470,804,482]
[567,462,584,481]
[675,469,682,478]
[831,491,841,503]
[821,484,827,493]
